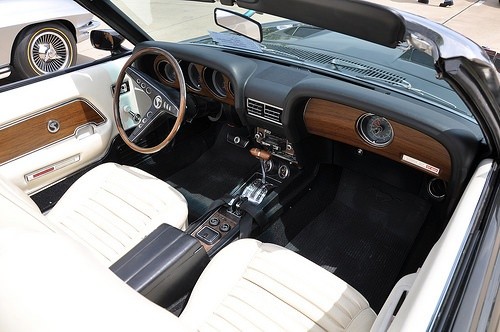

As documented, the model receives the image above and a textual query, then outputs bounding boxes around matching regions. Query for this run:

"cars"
[0,0,99,83]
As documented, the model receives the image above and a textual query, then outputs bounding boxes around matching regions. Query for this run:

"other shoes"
[440,1,454,7]
[418,0,429,4]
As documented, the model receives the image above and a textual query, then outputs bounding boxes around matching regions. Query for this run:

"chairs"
[0,160,382,332]
